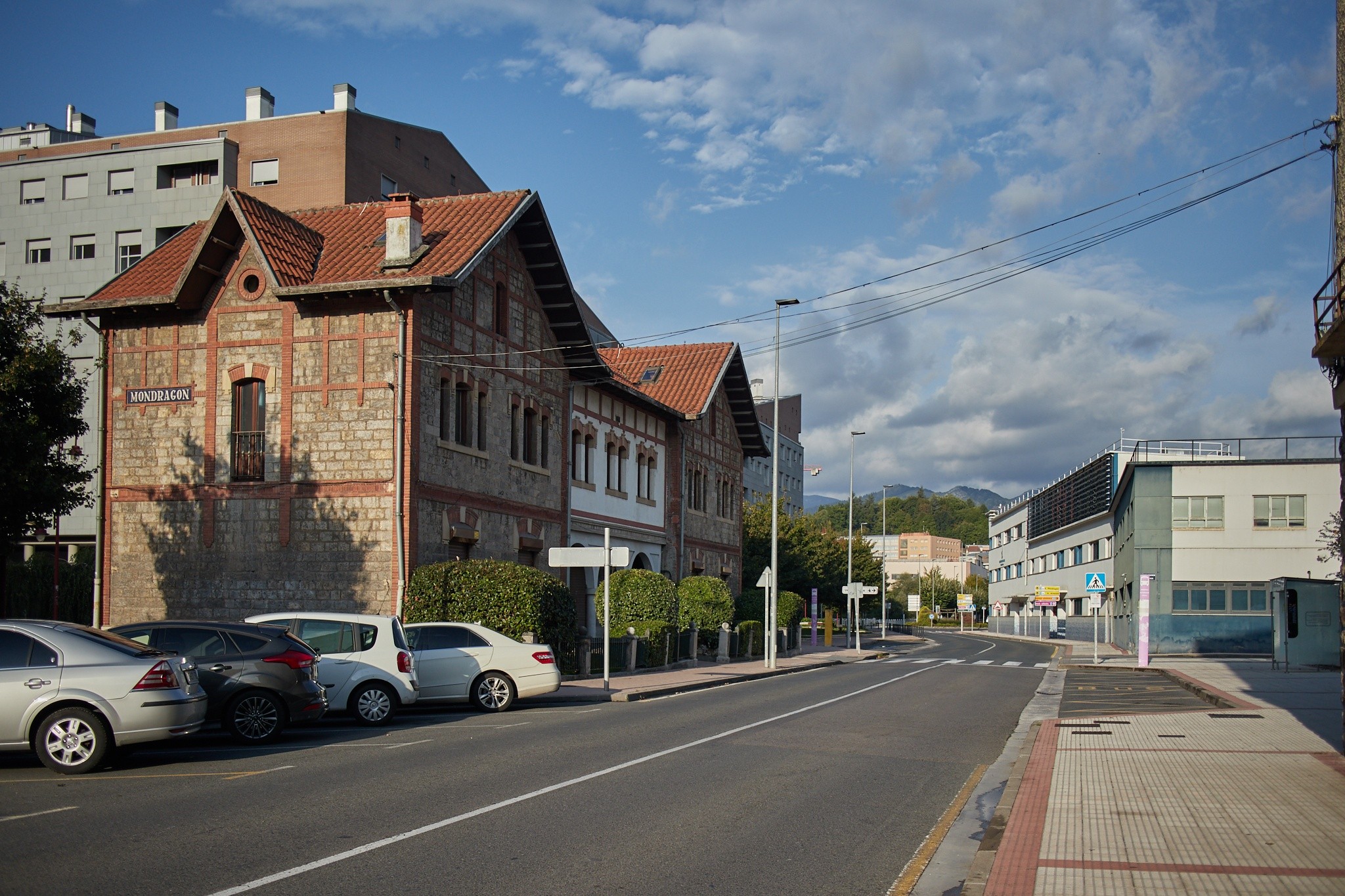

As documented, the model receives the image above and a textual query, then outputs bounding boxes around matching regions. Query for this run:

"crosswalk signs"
[1086,572,1106,592]
[970,605,976,611]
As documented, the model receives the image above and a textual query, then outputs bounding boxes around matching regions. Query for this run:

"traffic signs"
[1035,596,1060,601]
[957,594,973,612]
[1035,591,1060,596]
[1035,585,1060,591]
[1035,601,1057,606]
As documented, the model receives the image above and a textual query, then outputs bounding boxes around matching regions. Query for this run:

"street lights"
[958,541,963,594]
[919,554,924,595]
[932,559,936,612]
[954,566,957,579]
[976,574,980,597]
[848,431,867,654]
[882,484,893,638]
[768,298,799,669]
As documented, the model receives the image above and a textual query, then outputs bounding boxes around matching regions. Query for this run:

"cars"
[106,622,329,747]
[187,610,420,728]
[365,622,563,713]
[0,618,209,775]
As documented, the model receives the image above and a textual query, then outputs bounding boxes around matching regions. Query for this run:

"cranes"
[803,465,822,476]
